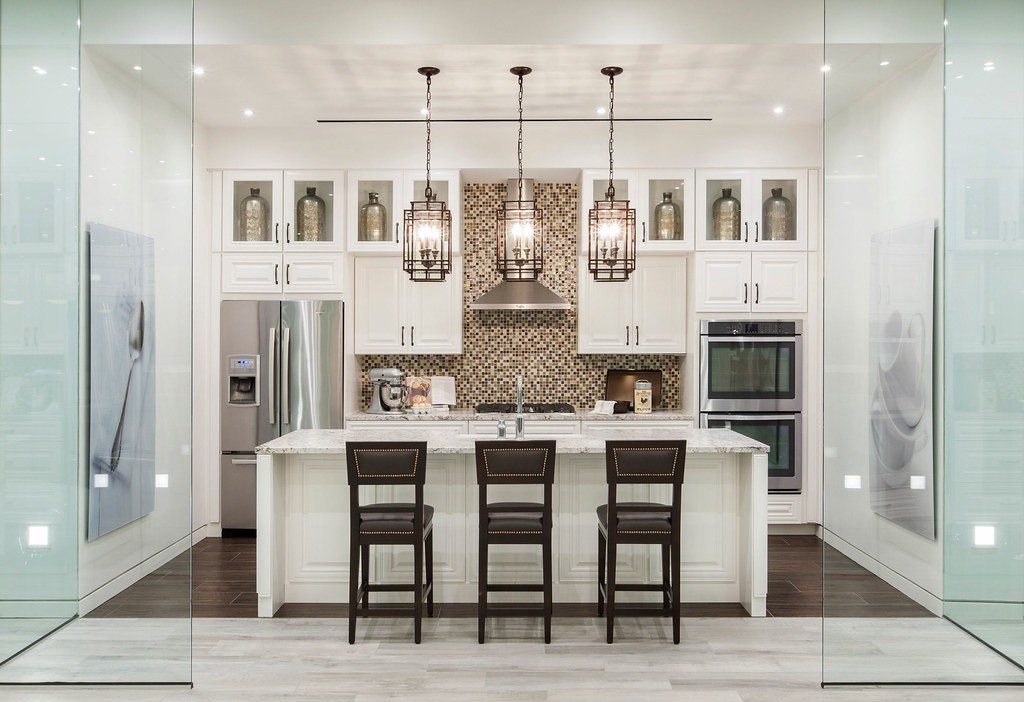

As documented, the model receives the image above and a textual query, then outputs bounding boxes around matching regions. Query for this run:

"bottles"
[359,191,387,241]
[241,187,268,242]
[712,188,740,241]
[654,192,681,240]
[764,188,792,240]
[297,186,326,242]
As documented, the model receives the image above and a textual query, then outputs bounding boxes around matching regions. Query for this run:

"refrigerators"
[219,300,345,537]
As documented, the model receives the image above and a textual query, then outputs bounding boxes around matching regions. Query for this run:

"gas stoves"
[473,401,577,414]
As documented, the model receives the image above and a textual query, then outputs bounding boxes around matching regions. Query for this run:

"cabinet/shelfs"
[222,170,463,355]
[577,168,807,354]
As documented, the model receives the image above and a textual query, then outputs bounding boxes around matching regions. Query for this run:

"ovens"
[700,318,803,494]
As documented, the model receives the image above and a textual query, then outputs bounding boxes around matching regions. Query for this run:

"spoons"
[110,301,144,471]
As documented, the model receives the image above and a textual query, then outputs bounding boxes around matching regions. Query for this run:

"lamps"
[403,67,452,282]
[496,66,544,283]
[588,66,636,282]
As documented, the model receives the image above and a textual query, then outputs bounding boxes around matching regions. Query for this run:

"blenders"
[364,369,409,415]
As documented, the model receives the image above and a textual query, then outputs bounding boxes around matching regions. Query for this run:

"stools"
[596,440,687,644]
[346,441,434,645]
[475,440,556,644]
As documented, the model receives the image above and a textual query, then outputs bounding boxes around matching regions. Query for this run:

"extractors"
[469,179,571,311]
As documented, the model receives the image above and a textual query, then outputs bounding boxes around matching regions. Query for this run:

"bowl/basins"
[614,400,632,413]
[381,386,409,410]
